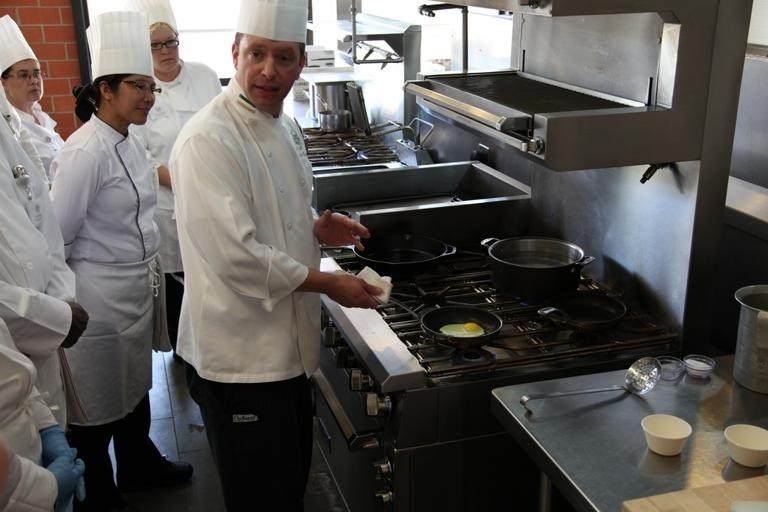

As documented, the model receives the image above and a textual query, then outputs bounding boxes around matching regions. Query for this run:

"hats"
[0,14,38,76]
[86,0,178,82]
[237,0,309,44]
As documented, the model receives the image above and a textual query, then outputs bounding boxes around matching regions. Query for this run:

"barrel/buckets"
[311,84,345,120]
[732,284,768,395]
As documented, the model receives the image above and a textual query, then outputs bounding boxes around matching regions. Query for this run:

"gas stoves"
[335,233,672,381]
[292,128,398,164]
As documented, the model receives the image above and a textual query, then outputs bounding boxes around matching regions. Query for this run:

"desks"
[490,352,764,512]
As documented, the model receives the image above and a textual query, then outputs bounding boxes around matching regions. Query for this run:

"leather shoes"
[167,461,194,478]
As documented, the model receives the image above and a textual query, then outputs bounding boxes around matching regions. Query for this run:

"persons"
[0,318,86,511]
[128,18,223,366]
[0,79,91,439]
[0,51,66,179]
[168,26,382,512]
[48,68,194,512]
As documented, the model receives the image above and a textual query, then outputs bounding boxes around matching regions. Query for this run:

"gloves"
[41,423,85,512]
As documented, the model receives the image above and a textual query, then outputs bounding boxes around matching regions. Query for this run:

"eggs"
[439,322,485,338]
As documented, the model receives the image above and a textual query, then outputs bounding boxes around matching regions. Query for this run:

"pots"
[481,235,596,297]
[316,94,351,132]
[321,234,458,277]
[389,300,505,349]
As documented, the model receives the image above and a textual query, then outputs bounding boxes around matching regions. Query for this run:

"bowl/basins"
[724,424,768,468]
[640,414,693,455]
[683,354,718,379]
[656,356,683,381]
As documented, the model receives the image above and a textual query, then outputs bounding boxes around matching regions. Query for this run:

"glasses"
[151,39,179,49]
[123,80,162,93]
[8,70,46,79]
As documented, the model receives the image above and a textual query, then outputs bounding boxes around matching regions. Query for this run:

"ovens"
[310,330,391,511]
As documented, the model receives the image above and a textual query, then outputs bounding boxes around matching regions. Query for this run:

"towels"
[356,266,394,304]
